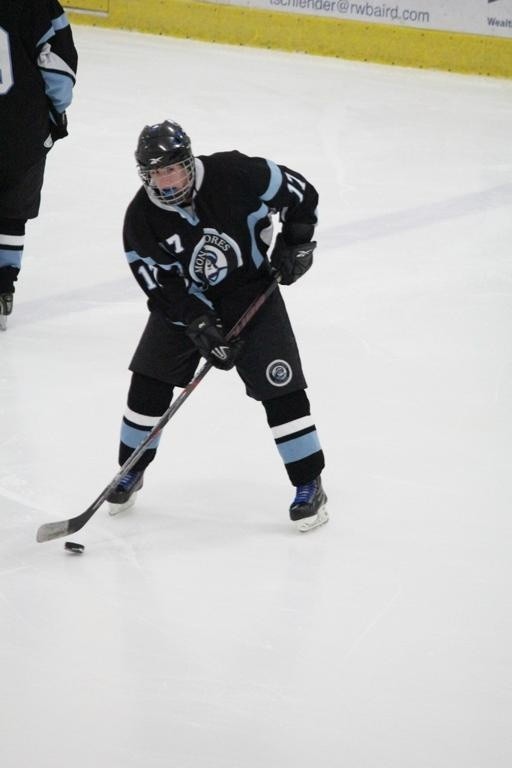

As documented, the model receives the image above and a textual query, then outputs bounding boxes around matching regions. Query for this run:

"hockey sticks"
[37,271,282,542]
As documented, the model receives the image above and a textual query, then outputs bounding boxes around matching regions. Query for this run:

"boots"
[289,474,328,521]
[0,292,13,316]
[106,466,143,504]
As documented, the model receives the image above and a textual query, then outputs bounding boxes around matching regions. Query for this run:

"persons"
[106,120,327,522]
[0,0,79,315]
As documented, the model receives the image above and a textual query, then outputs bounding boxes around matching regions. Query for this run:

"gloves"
[185,314,245,371]
[270,221,317,285]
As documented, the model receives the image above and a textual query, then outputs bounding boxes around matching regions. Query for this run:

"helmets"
[134,119,193,172]
